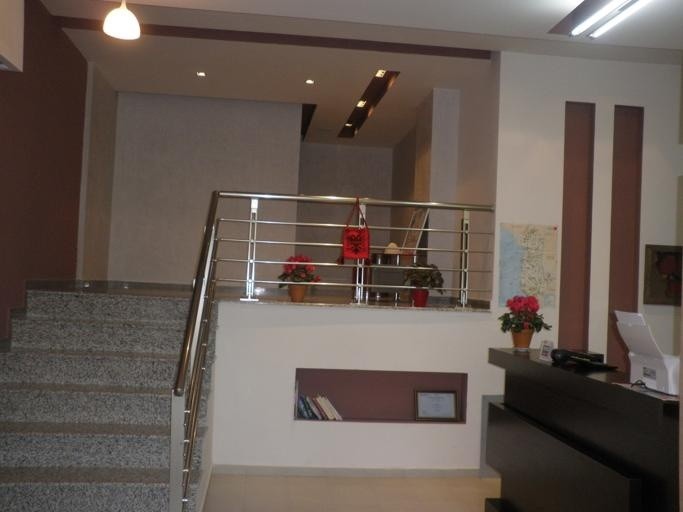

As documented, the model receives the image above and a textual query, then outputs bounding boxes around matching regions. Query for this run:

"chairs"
[353,249,417,308]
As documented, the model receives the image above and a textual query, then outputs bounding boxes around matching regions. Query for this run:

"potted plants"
[404,260,446,307]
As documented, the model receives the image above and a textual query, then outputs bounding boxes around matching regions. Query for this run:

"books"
[298,393,342,421]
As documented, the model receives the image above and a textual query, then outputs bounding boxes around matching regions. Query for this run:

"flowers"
[498,294,557,333]
[278,256,322,289]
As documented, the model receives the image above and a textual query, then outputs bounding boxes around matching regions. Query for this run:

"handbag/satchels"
[342,228,370,260]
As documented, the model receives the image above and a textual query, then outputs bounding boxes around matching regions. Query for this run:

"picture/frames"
[643,242,682,306]
[414,389,460,421]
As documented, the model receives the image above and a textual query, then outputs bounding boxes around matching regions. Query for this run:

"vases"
[287,284,308,303]
[511,324,534,348]
